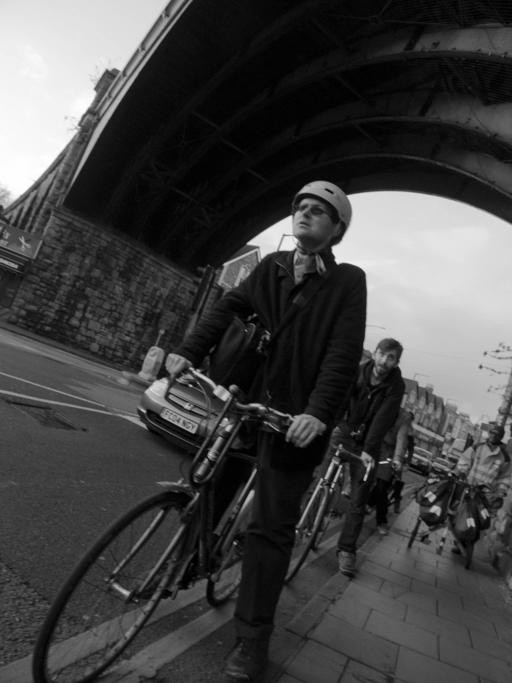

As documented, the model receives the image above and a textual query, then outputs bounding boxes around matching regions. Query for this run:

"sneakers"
[335,547,359,578]
[375,521,389,535]
[222,631,273,681]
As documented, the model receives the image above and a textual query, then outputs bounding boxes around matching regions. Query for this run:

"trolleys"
[408,472,493,569]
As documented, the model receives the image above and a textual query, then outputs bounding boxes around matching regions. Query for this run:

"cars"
[406,446,458,476]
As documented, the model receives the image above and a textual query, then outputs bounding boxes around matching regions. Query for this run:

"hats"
[488,425,504,439]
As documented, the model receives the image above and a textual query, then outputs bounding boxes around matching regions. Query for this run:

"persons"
[450,423,512,555]
[162,177,369,680]
[375,406,414,535]
[334,337,405,575]
[394,410,415,514]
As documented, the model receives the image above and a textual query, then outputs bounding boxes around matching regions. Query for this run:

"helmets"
[290,178,354,243]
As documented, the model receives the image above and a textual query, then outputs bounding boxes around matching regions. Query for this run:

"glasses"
[294,202,335,220]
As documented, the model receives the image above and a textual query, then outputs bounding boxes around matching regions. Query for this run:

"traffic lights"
[189,264,213,313]
